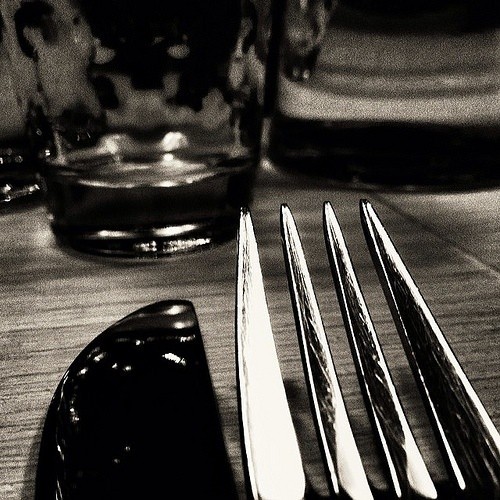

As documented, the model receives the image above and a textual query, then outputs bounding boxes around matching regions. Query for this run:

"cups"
[0,28,42,216]
[0,0,276,260]
[267,1,500,195]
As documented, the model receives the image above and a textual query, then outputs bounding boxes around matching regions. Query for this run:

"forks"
[232,198,500,500]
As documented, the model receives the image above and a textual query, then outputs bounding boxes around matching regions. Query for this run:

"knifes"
[33,297,241,499]
